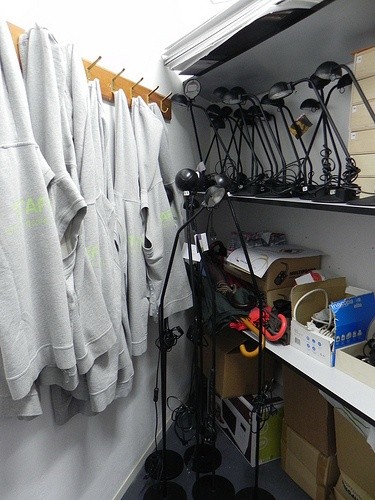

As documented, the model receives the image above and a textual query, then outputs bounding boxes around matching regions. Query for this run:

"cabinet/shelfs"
[183,0,375,427]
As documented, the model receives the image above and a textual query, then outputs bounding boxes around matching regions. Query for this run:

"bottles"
[227,231,260,251]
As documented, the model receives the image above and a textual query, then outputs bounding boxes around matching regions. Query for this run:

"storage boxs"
[224,249,321,290]
[290,277,375,367]
[207,379,284,468]
[231,275,293,308]
[333,408,375,500]
[282,364,336,457]
[280,418,340,500]
[334,339,375,389]
[198,323,274,399]
[333,473,375,500]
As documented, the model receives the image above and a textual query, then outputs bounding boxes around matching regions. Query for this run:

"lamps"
[143,60,375,500]
